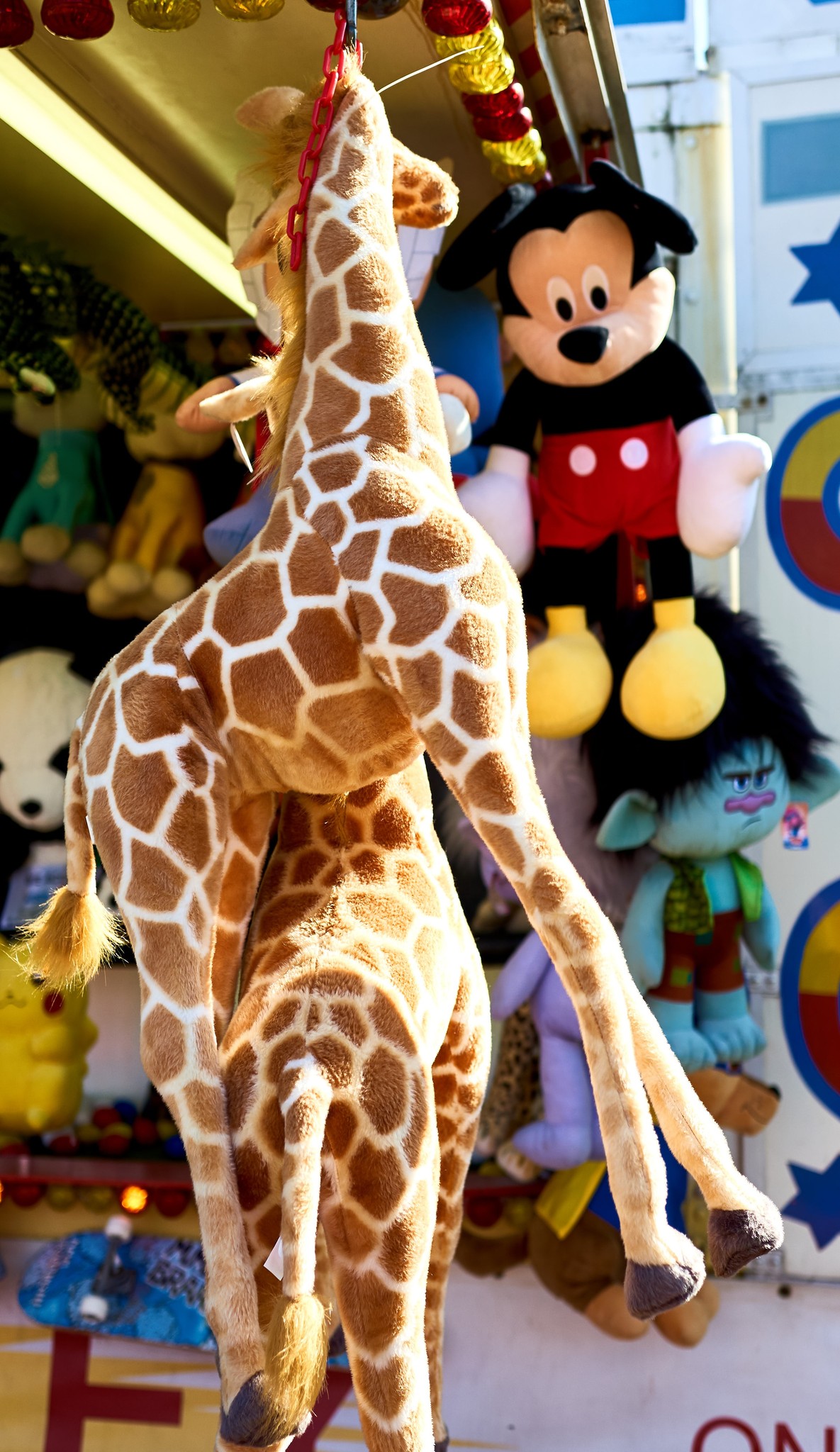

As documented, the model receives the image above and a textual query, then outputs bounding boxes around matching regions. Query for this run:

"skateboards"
[17,1214,353,1370]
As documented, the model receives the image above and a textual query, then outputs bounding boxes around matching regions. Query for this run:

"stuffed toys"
[435,159,775,740]
[583,584,840,1073]
[0,74,840,1452]
